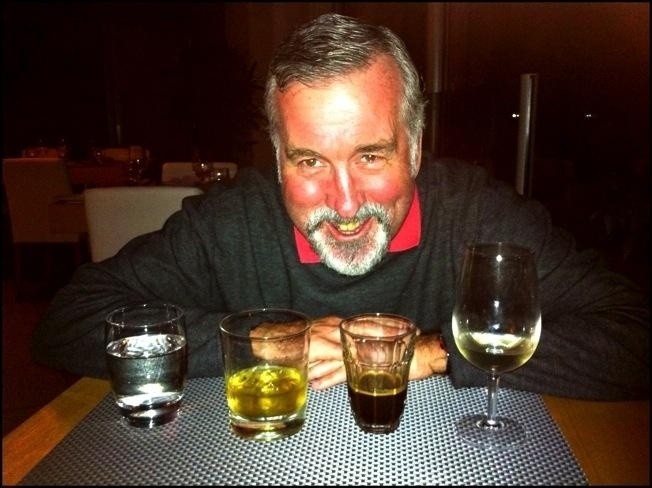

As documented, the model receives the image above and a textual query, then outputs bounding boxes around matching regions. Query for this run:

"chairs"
[1,156,238,269]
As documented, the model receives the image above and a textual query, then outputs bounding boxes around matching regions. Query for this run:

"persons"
[29,11,650,404]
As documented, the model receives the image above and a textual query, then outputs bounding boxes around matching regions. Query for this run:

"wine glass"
[451,241,543,451]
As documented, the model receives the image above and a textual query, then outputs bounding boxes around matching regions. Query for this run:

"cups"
[339,312,417,435]
[127,151,228,187]
[104,301,188,428]
[218,308,313,443]
